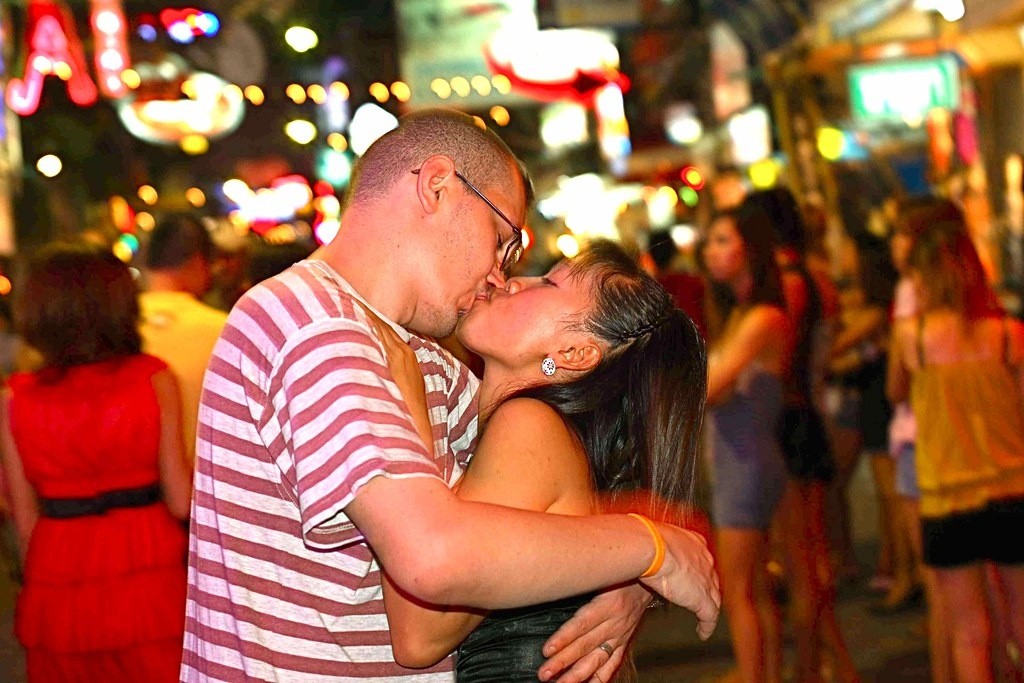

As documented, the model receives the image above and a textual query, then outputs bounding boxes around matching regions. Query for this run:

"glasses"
[411,168,524,271]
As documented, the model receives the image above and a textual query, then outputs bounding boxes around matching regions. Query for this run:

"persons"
[180,106,722,683]
[344,237,706,683]
[0,210,317,683]
[642,185,1024,683]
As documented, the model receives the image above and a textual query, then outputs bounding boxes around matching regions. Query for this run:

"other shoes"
[872,586,922,616]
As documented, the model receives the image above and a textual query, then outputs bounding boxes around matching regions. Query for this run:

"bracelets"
[628,513,666,577]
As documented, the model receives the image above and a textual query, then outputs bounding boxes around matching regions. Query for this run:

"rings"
[601,643,614,655]
